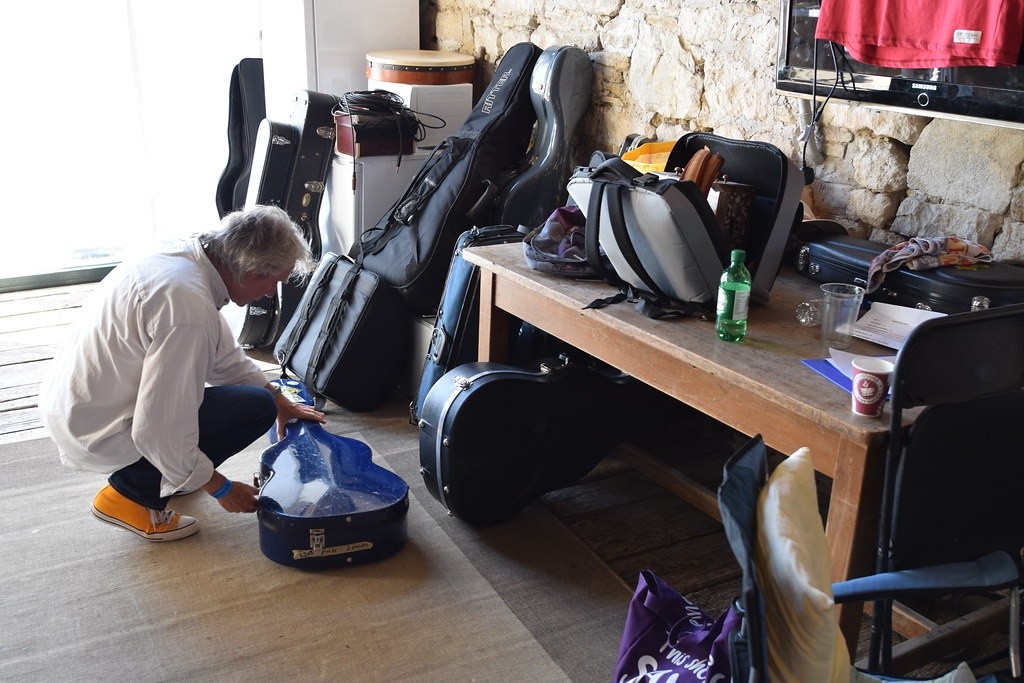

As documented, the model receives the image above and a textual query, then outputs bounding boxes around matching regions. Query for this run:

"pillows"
[752,445,851,683]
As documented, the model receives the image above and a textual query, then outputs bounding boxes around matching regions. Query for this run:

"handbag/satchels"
[613,569,749,683]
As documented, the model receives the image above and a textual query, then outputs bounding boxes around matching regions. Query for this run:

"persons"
[36,205,327,542]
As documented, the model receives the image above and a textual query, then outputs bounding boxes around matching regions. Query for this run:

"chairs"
[868,304,1023,682]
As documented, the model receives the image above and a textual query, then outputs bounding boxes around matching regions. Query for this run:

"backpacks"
[520,131,662,283]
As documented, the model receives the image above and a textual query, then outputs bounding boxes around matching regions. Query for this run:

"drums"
[364,43,477,91]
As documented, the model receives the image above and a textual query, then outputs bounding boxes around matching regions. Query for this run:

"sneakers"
[90,483,199,542]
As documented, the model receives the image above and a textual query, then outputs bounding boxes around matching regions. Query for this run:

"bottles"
[715,250,752,342]
[795,298,823,327]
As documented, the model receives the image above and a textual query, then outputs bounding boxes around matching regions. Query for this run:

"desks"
[460,241,1024,683]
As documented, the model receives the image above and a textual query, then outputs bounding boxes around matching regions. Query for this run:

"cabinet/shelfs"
[260,0,443,257]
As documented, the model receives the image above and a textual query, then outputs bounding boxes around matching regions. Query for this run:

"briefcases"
[566,129,805,321]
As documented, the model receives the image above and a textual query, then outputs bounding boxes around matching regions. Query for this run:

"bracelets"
[272,386,281,401]
[211,477,232,500]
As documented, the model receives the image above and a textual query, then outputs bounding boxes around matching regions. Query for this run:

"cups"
[820,283,866,349]
[850,357,895,417]
